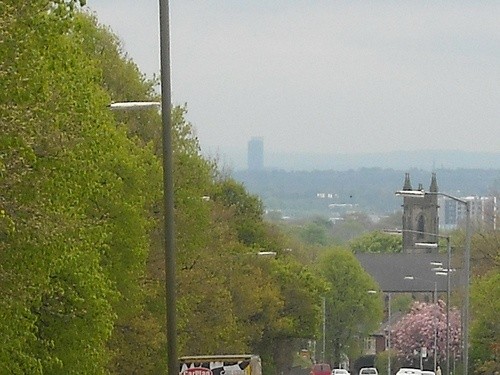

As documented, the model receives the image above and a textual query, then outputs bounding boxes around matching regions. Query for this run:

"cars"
[309,363,333,375]
[331,368,350,375]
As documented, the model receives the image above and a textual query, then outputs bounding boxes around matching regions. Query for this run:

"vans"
[359,367,378,375]
[177,355,262,375]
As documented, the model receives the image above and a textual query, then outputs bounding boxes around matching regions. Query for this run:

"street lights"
[367,187,471,375]
